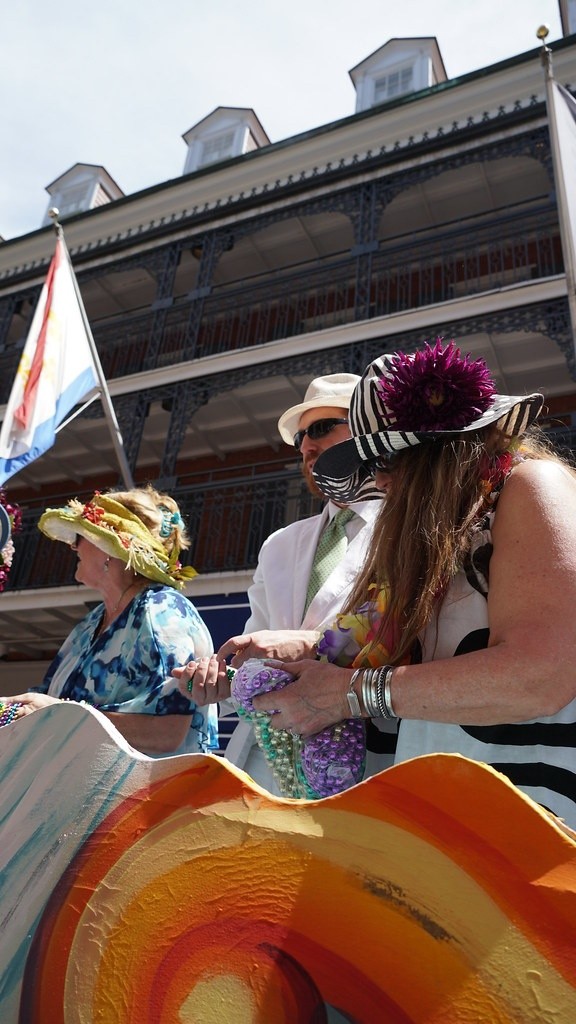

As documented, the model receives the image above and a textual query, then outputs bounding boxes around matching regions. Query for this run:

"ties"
[302,507,355,624]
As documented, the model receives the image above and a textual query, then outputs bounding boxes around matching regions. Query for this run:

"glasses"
[292,418,348,451]
[74,533,85,548]
[360,450,405,475]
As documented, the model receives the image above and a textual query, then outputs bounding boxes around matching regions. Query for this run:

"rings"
[289,729,301,741]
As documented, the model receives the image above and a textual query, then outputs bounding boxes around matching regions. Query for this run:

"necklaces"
[94,574,146,640]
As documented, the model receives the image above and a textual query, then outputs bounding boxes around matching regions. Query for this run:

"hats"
[277,372,363,445]
[349,337,546,462]
[36,488,200,590]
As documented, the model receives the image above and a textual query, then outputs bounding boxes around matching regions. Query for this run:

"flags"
[0,232,102,489]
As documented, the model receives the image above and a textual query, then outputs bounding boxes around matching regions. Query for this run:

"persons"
[0,482,221,758]
[170,371,391,801]
[252,352,576,839]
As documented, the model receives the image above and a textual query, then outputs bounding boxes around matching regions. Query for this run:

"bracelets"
[60,697,100,711]
[346,665,366,720]
[363,665,397,720]
